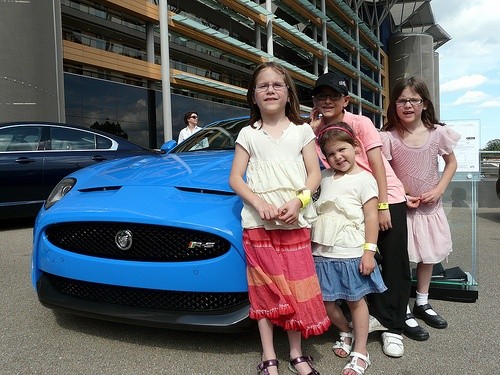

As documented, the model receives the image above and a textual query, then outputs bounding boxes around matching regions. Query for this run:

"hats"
[313,72,349,95]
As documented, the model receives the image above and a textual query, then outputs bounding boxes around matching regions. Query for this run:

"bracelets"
[377,202,389,209]
[297,190,312,208]
[364,243,380,254]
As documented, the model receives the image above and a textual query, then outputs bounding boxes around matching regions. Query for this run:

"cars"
[31,115,321,336]
[0,123,157,221]
[481,161,499,178]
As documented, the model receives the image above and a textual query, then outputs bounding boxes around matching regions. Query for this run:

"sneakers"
[382,332,404,357]
[349,314,388,334]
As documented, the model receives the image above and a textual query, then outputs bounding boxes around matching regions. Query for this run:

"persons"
[177,111,210,151]
[308,121,388,375]
[307,72,408,358]
[377,77,461,341]
[228,61,331,375]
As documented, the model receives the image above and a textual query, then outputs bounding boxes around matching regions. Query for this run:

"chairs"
[9,140,32,150]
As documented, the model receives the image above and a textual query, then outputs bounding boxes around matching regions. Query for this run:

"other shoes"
[402,314,430,340]
[413,301,448,328]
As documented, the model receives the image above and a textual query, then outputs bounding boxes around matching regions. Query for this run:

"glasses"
[396,98,423,108]
[190,115,198,119]
[255,81,287,92]
[315,92,344,103]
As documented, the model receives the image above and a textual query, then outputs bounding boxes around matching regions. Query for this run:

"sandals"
[288,356,321,375]
[257,360,279,375]
[333,331,355,357]
[341,351,371,375]
